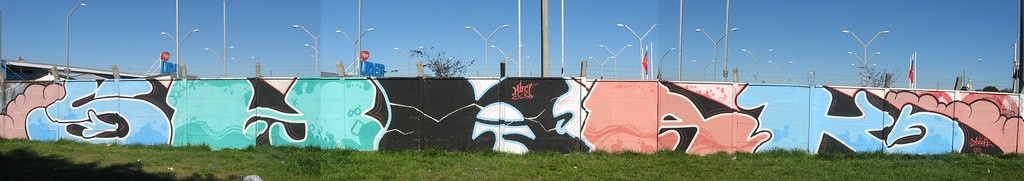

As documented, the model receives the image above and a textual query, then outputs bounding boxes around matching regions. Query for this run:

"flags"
[908,54,915,85]
[642,50,648,75]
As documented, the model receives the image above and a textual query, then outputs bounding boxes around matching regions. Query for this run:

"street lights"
[161,29,199,79]
[589,56,615,79]
[204,45,234,78]
[741,48,773,84]
[464,24,509,77]
[335,27,375,78]
[293,24,323,78]
[598,45,632,81]
[660,47,676,81]
[767,60,793,79]
[692,59,715,81]
[66,3,87,80]
[695,27,738,82]
[671,67,673,68]
[616,23,659,81]
[491,45,523,77]
[842,29,890,88]
